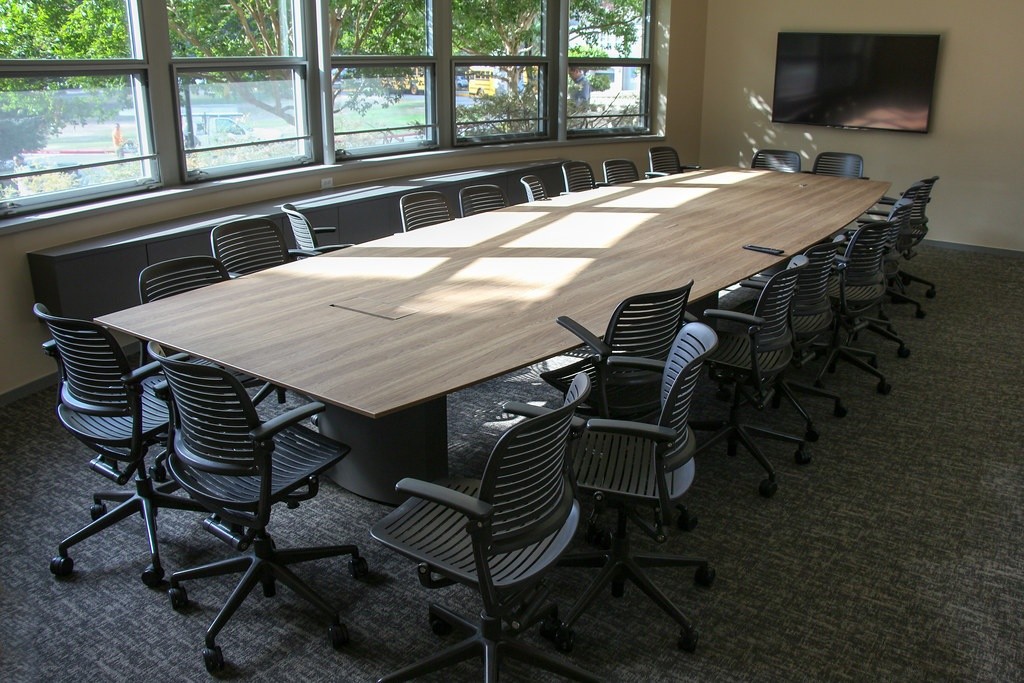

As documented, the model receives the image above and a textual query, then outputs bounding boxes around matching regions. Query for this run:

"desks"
[93,166,890,508]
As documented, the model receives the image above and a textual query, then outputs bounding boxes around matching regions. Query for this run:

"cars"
[456,75,468,89]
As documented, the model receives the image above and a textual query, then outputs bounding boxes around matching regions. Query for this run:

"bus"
[469,65,543,100]
[334,66,426,95]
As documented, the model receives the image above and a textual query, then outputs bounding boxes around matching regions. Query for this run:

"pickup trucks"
[183,116,256,147]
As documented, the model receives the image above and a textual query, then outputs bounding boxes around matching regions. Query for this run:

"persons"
[115,121,123,156]
[12,155,33,195]
[568,64,591,127]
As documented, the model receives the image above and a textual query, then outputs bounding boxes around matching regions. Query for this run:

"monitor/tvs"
[772,32,940,134]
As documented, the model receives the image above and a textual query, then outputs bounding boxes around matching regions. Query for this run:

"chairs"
[496,321,719,648]
[533,167,941,544]
[33,299,275,588]
[367,367,618,682]
[139,145,684,402]
[145,334,368,676]
